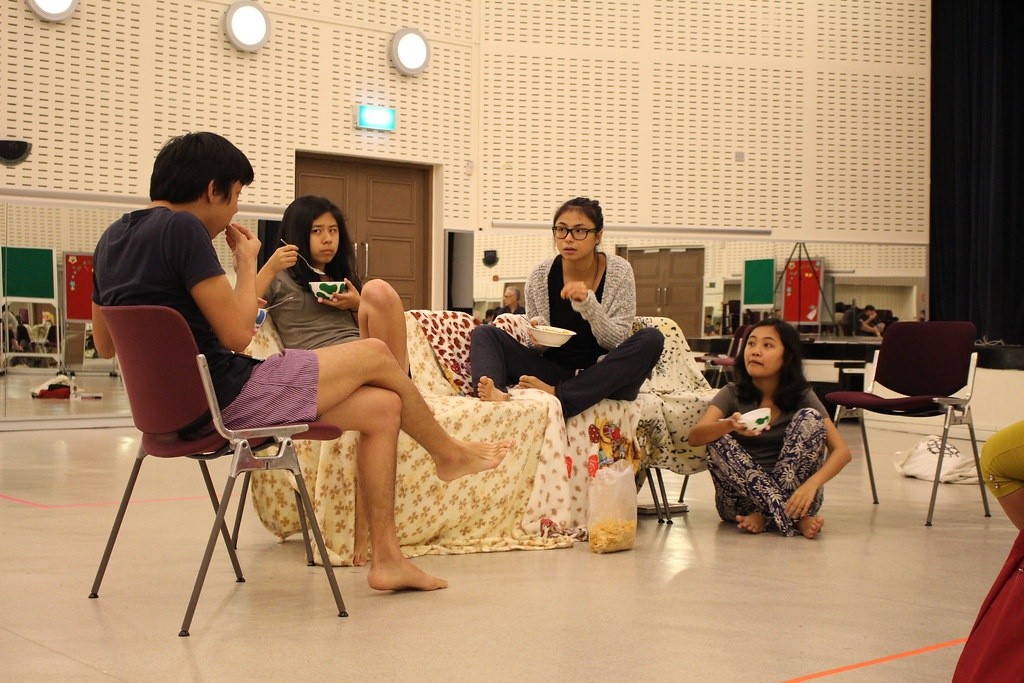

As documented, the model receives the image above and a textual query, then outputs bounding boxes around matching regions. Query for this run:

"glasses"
[504,295,514,298]
[552,226,598,240]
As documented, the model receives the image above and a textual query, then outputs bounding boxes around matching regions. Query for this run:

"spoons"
[280,238,325,275]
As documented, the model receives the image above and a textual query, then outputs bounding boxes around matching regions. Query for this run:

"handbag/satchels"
[894,435,982,485]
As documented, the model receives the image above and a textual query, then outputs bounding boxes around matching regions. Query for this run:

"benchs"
[246,309,719,562]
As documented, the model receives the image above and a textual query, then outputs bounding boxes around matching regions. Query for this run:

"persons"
[836,300,929,337]
[469,199,664,419]
[688,319,852,540]
[256,194,411,567]
[90,132,509,591]
[952,419,1024,683]
[705,314,721,334]
[2,304,56,356]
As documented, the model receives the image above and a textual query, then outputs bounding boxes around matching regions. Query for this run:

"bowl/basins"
[737,407,771,431]
[526,324,577,347]
[254,308,269,327]
[309,282,347,301]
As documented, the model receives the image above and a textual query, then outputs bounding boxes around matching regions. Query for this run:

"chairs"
[84,306,350,638]
[825,320,991,532]
[701,325,753,389]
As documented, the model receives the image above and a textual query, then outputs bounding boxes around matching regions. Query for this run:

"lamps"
[389,27,430,75]
[25,0,78,24]
[223,2,272,53]
